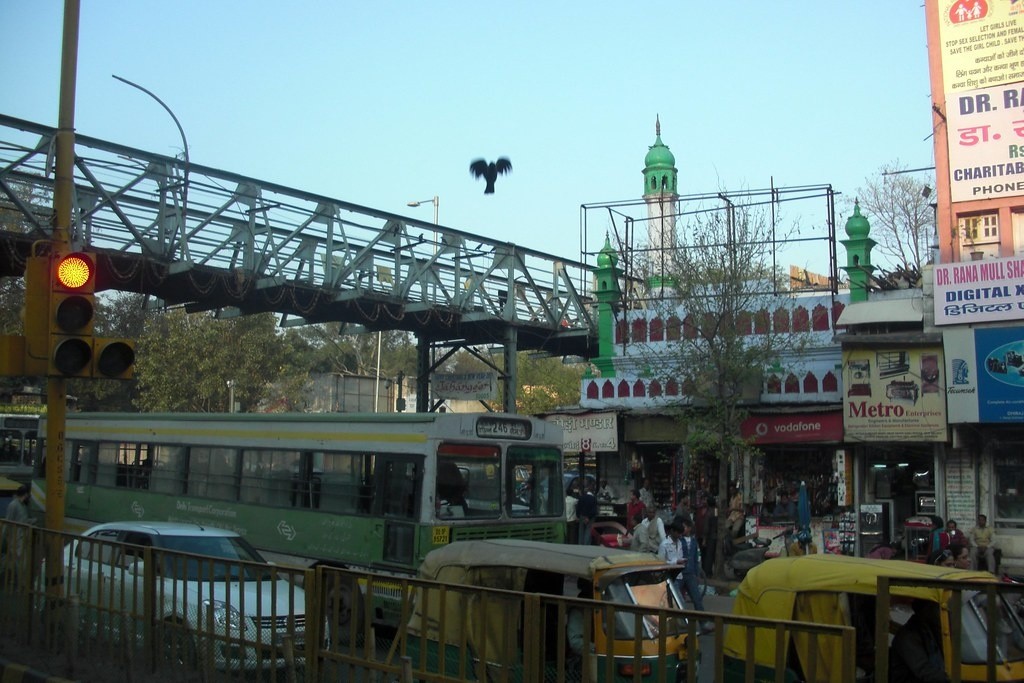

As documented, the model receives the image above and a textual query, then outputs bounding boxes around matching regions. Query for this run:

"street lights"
[406,196,439,301]
[111,73,189,261]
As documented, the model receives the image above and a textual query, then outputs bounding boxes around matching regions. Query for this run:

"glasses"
[943,561,956,567]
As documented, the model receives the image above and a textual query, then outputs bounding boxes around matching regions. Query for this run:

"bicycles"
[681,566,707,604]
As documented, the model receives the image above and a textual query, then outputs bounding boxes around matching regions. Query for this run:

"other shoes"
[701,621,716,636]
[696,632,700,636]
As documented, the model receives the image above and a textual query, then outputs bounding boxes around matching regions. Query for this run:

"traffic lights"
[49,250,135,379]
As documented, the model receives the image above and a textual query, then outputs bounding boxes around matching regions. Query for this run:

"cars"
[34,521,330,683]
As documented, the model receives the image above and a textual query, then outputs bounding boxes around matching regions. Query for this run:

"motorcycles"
[398,536,715,683]
[724,552,1024,683]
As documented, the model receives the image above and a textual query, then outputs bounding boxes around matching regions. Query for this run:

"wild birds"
[469,157,513,194]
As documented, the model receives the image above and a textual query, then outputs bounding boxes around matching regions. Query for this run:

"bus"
[0,413,41,524]
[28,410,568,649]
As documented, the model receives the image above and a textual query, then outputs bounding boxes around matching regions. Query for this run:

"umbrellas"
[797,481,812,555]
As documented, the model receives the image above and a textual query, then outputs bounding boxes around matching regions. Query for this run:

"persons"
[868,543,898,559]
[726,482,745,536]
[623,515,649,554]
[889,599,953,683]
[521,480,542,512]
[642,508,666,554]
[576,484,597,545]
[597,478,615,498]
[671,492,717,579]
[627,489,646,528]
[677,517,715,634]
[657,523,700,636]
[0,486,38,588]
[969,514,997,575]
[926,517,950,566]
[639,479,656,508]
[772,491,796,521]
[568,579,594,655]
[564,488,579,544]
[945,521,969,559]
